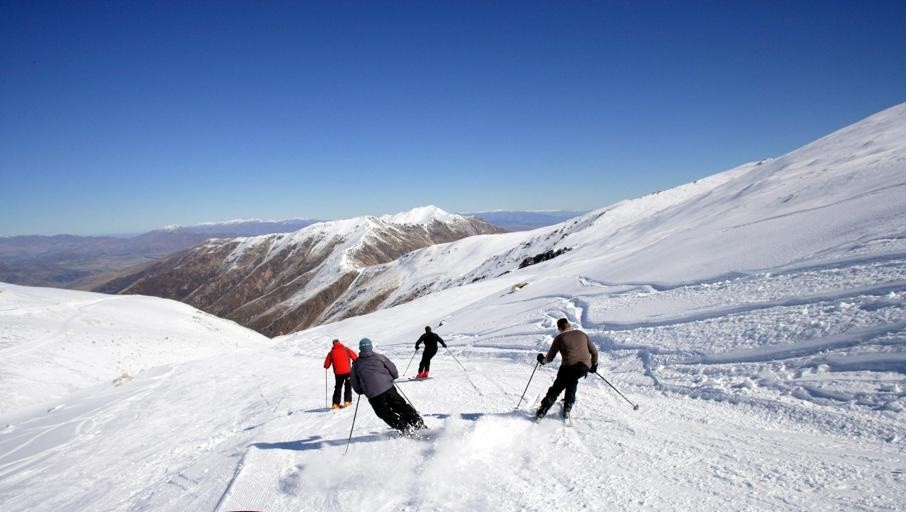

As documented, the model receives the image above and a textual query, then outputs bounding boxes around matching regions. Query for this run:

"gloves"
[537,353,545,365]
[589,363,598,373]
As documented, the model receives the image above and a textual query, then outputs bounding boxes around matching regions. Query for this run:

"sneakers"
[331,401,353,411]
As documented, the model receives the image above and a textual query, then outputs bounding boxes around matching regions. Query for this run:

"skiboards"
[560,399,573,428]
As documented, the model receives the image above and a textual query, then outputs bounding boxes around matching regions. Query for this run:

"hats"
[359,337,373,352]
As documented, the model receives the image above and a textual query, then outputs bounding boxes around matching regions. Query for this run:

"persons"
[323,339,359,409]
[534,317,598,418]
[351,337,428,435]
[415,326,447,379]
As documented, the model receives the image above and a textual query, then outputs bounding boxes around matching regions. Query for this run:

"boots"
[413,371,429,380]
[561,401,573,418]
[535,405,549,418]
